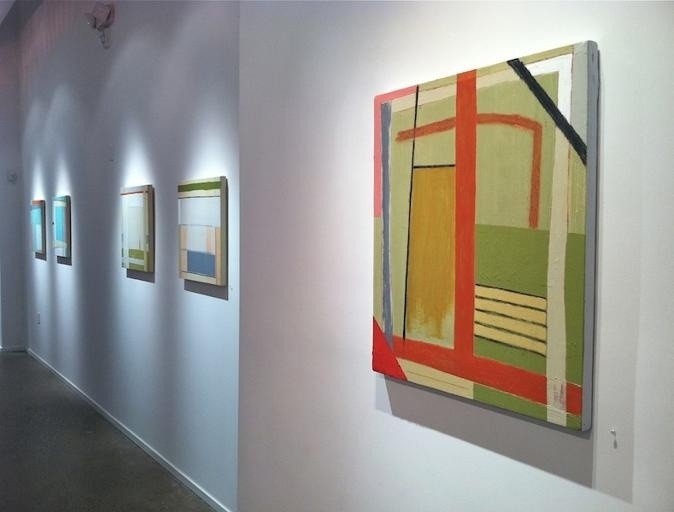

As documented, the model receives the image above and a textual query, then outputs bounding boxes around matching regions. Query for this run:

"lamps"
[84,1,115,33]
